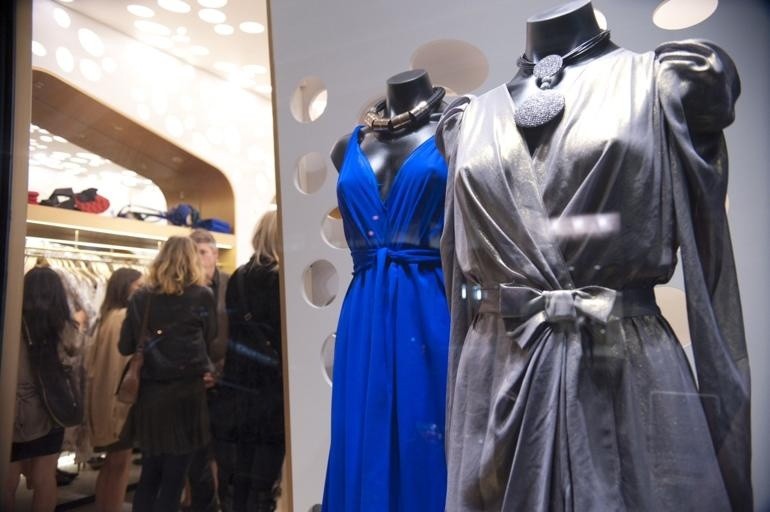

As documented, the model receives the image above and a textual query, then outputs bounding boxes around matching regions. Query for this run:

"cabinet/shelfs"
[15,66,237,512]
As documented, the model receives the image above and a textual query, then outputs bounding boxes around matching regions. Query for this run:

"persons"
[84,268,144,512]
[8,266,79,511]
[209,209,286,512]
[440,1,755,512]
[190,227,230,500]
[317,70,454,512]
[117,237,218,512]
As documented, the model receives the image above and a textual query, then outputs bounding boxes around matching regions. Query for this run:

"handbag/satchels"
[39,358,80,427]
[116,345,151,403]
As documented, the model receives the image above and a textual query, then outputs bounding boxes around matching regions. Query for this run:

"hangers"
[25,254,133,280]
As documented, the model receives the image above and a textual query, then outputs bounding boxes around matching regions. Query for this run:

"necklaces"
[361,86,447,133]
[513,27,617,126]
